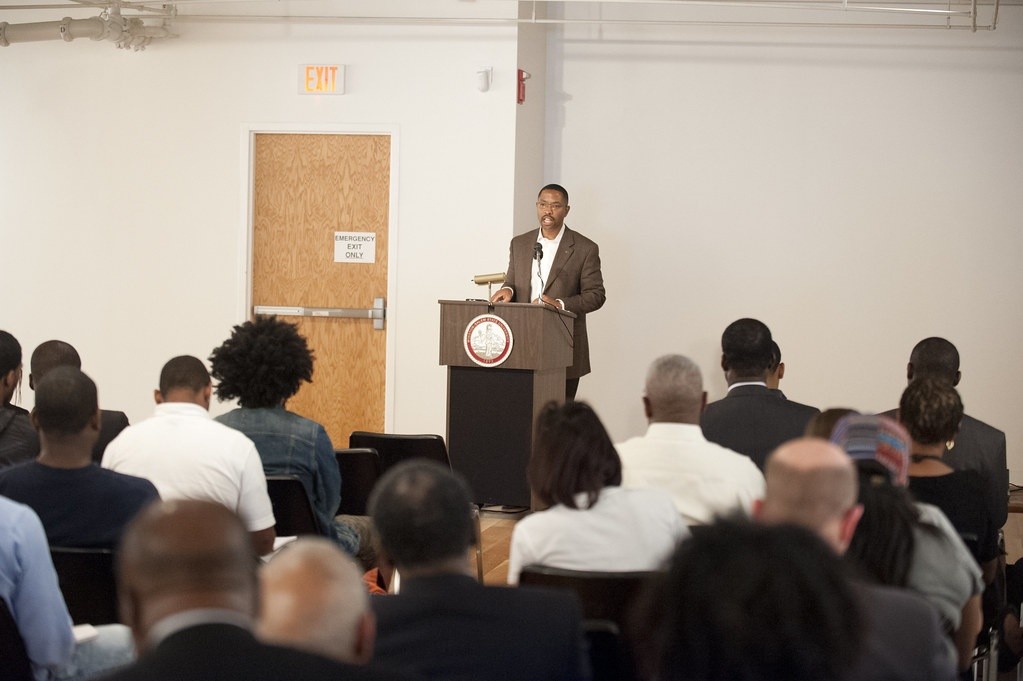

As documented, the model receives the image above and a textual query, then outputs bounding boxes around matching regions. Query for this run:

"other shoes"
[997,604,1021,673]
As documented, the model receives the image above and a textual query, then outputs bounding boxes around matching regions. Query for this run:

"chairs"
[0,597,39,680]
[40,546,125,632]
[347,430,485,583]
[517,560,653,681]
[334,448,380,517]
[958,535,1001,681]
[265,478,325,541]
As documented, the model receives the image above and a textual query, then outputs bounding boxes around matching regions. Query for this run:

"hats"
[829,413,912,489]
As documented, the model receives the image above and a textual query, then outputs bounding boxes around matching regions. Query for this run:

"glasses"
[539,202,562,208]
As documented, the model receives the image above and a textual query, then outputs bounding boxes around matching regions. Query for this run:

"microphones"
[532,242,544,260]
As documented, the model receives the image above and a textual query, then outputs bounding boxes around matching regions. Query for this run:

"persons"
[0,314,392,681]
[367,460,592,681]
[507,318,1023,681]
[489,184,606,401]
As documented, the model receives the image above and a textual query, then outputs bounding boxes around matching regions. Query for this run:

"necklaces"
[910,452,944,465]
